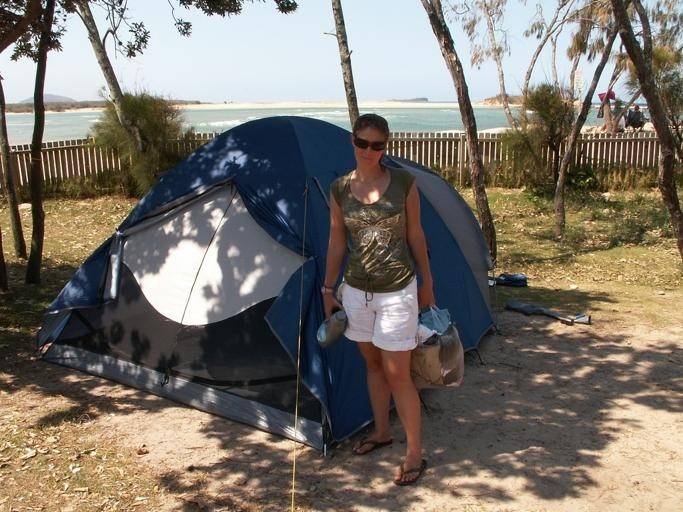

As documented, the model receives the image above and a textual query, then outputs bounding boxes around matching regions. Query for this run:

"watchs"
[320,285,334,295]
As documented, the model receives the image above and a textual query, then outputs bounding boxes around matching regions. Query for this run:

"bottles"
[316,308,347,349]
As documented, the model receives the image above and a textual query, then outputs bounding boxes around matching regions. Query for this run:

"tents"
[33,116,500,457]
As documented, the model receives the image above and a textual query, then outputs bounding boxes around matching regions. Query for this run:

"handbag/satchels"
[412,324,465,390]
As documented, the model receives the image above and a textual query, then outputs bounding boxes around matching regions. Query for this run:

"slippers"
[352,437,392,454]
[393,459,425,485]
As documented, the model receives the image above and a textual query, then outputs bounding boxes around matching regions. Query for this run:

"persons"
[612,100,647,132]
[320,114,435,486]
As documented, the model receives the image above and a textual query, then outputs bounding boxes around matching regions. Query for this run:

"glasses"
[354,135,385,151]
